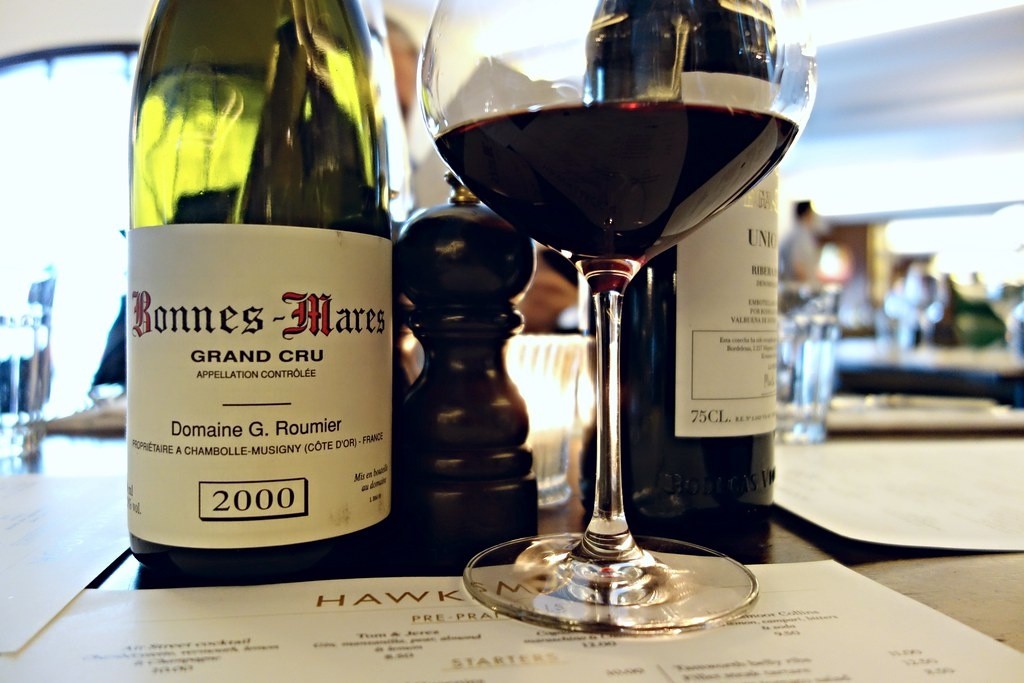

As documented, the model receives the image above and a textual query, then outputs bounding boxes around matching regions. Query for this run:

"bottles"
[130,0,394,578]
[584,0,779,560]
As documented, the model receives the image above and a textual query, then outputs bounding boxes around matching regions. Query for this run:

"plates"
[789,395,1024,430]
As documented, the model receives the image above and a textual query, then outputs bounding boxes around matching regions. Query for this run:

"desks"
[786,394,1024,440]
[810,337,1024,406]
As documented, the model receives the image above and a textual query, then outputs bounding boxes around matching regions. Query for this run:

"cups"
[775,284,842,446]
[875,298,917,352]
[503,334,586,510]
[0,265,55,475]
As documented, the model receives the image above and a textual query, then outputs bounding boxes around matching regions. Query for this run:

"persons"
[885,257,960,347]
[776,201,823,321]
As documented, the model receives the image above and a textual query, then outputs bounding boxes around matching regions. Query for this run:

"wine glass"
[418,0,818,640]
